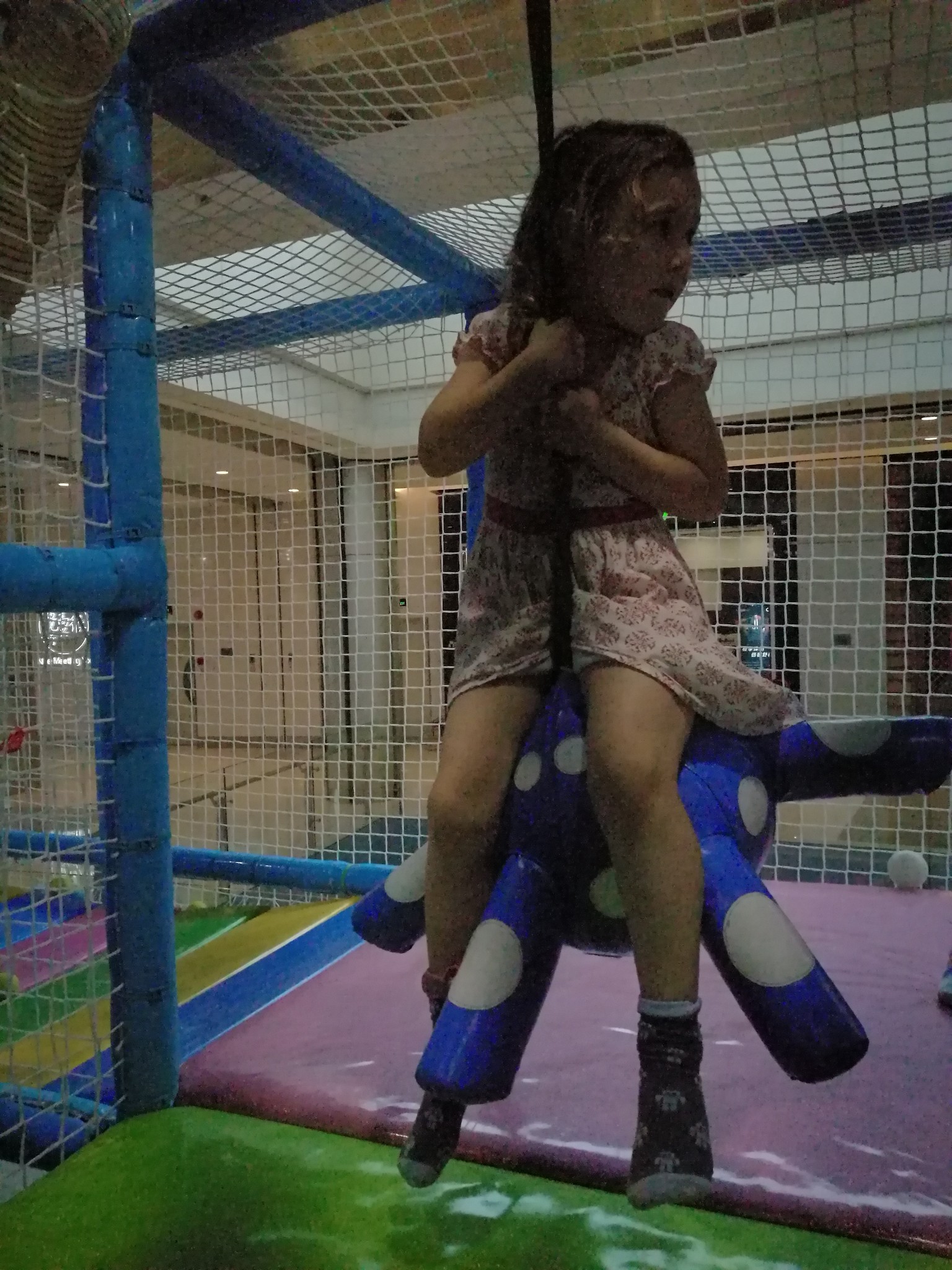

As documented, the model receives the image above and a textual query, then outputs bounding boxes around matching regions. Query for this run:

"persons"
[413,120,810,1027]
